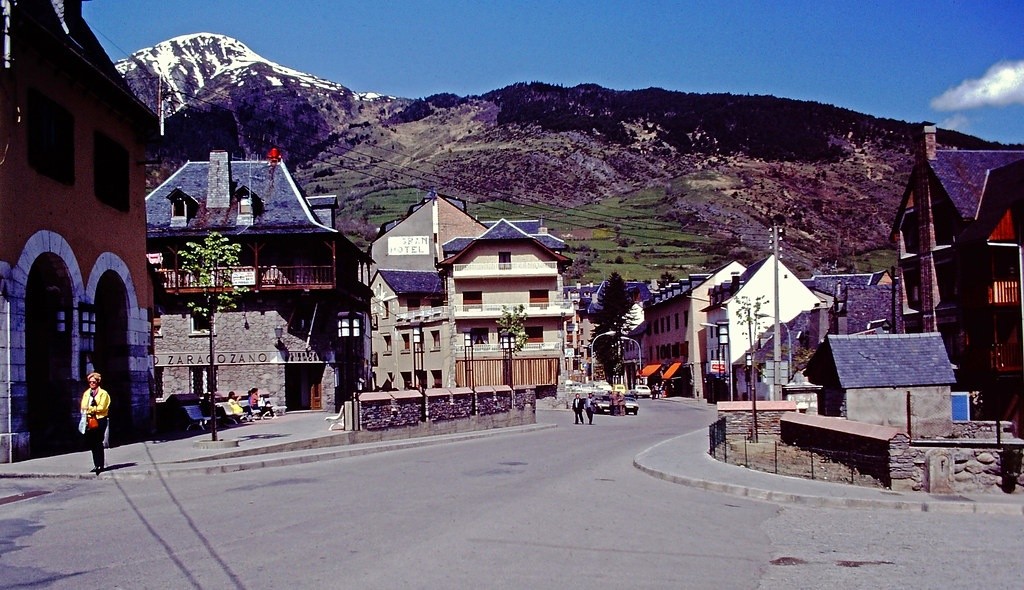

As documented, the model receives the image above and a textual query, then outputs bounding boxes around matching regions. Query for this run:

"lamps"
[274,326,285,351]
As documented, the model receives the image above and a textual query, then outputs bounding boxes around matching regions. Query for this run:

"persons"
[609,392,627,416]
[572,393,584,424]
[201,393,229,428]
[79,372,111,475]
[651,381,674,400]
[227,388,280,420]
[585,393,597,424]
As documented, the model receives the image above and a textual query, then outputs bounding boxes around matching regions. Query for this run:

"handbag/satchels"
[88,418,98,428]
[258,395,265,407]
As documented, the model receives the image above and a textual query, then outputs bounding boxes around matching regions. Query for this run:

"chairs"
[325,405,344,430]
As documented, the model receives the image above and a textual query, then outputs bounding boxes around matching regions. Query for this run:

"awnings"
[640,364,661,376]
[662,363,681,379]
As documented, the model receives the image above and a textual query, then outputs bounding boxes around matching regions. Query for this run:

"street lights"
[754,312,792,382]
[620,336,641,370]
[769,221,784,400]
[591,331,617,381]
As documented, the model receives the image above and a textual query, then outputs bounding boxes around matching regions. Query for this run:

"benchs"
[182,397,287,431]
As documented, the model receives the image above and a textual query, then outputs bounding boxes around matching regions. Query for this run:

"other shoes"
[90,466,103,473]
[272,413,279,419]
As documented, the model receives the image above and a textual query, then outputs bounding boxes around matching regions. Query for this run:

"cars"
[592,395,610,415]
[563,380,652,398]
[624,397,639,415]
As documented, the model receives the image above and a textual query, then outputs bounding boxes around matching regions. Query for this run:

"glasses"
[89,381,97,384]
